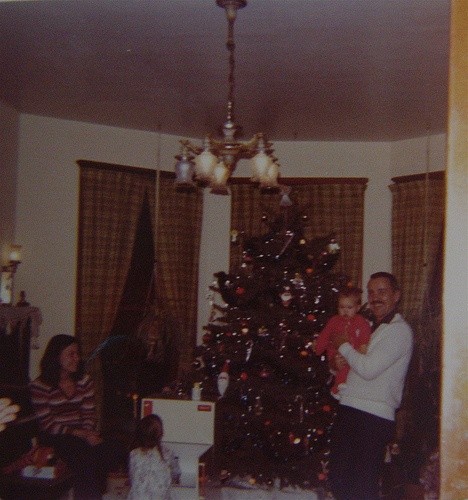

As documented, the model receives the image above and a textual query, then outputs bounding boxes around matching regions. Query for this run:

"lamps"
[0,244,23,274]
[174,0,283,197]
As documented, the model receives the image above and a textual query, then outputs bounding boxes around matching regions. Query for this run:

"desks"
[0,307,43,468]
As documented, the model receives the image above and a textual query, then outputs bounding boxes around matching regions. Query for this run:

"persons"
[329,272,413,500]
[311,286,371,401]
[30,335,107,500]
[127,414,181,500]
